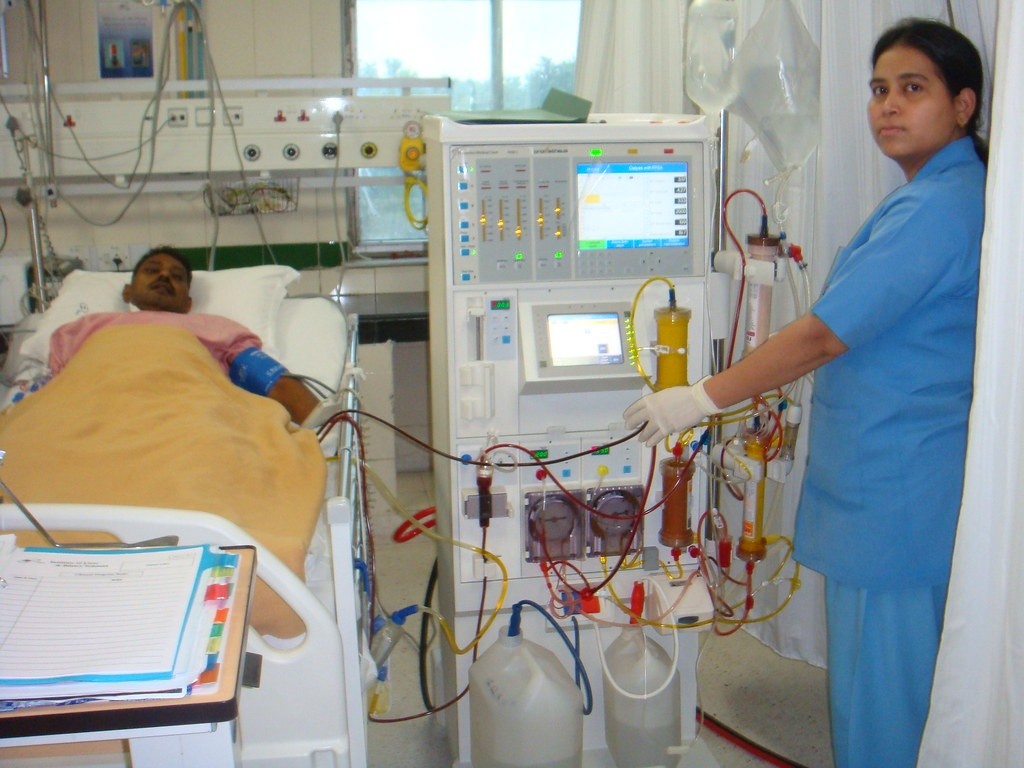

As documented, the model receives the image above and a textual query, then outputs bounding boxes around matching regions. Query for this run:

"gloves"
[623,375,727,447]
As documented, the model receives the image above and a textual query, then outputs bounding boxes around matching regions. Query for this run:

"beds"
[0,293,368,768]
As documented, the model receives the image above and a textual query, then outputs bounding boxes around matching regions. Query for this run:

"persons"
[623,19,988,768]
[49,245,325,425]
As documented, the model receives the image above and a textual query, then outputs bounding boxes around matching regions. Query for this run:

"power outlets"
[167,109,188,128]
[223,107,244,126]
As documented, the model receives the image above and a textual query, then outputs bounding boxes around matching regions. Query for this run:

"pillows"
[18,264,301,361]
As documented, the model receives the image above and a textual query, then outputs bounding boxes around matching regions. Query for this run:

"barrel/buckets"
[601,625,681,768]
[468,625,584,768]
